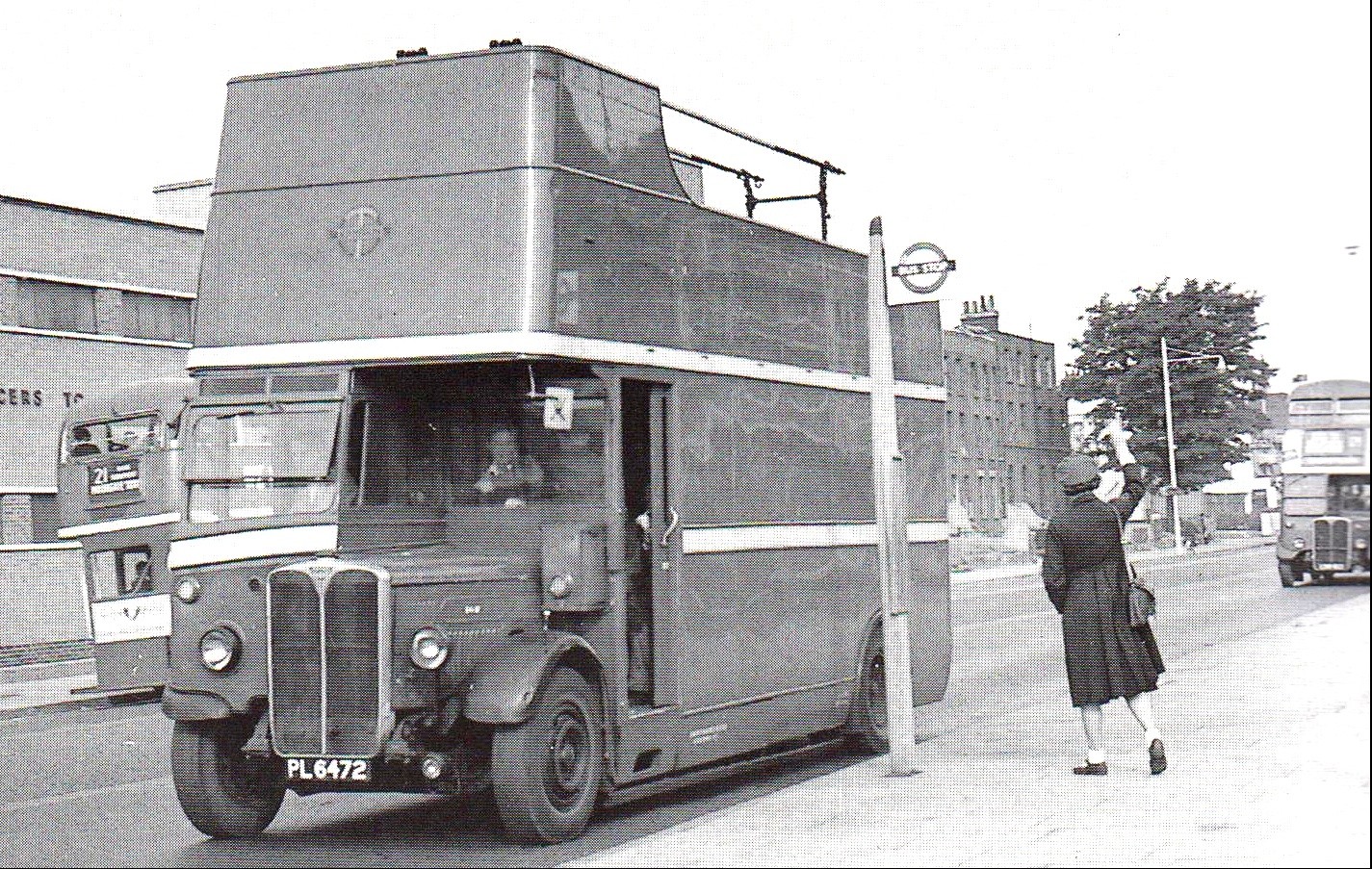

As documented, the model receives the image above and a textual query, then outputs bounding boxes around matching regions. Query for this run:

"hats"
[1055,454,1101,492]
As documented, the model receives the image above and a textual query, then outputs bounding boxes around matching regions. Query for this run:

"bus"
[1272,379,1370,590]
[53,374,194,708]
[155,37,957,852]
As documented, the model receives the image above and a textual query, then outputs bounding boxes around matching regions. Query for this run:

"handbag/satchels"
[1128,583,1158,627]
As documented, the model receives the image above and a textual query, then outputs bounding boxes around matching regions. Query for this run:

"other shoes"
[1073,760,1108,776]
[1148,739,1167,775]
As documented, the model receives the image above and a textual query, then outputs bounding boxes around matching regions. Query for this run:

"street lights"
[1159,335,1228,557]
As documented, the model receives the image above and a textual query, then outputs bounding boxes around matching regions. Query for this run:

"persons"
[1040,413,1169,777]
[130,560,150,592]
[473,422,545,508]
[69,426,100,457]
